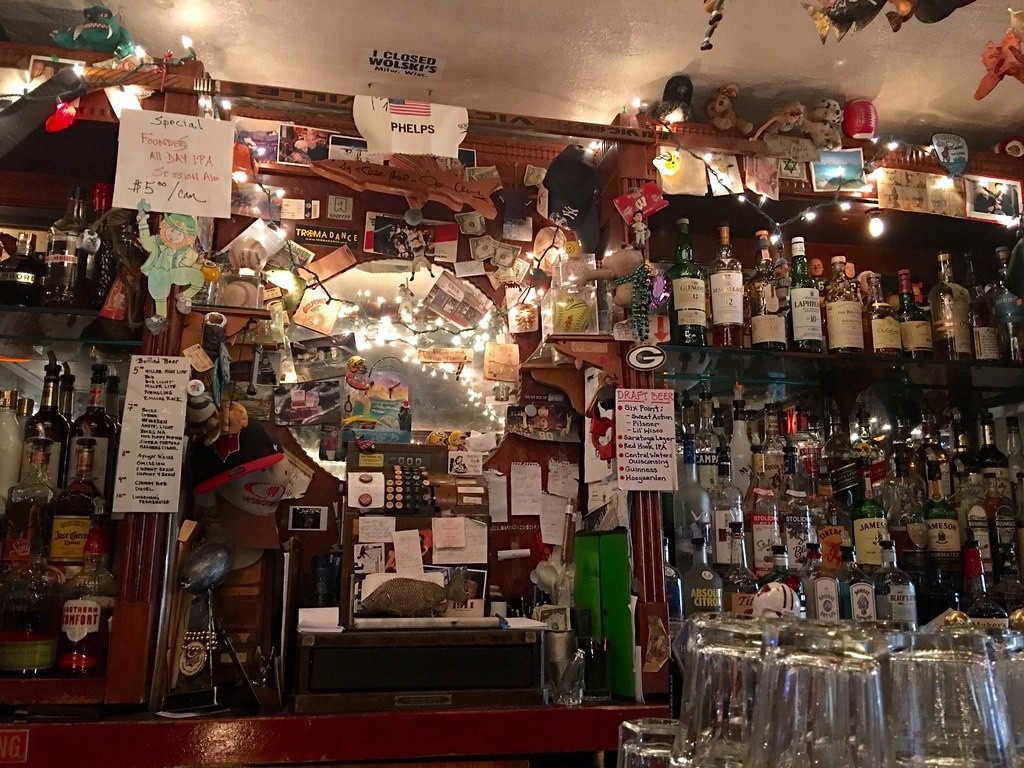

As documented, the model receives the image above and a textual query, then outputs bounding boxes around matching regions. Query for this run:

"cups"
[573,634,611,701]
[550,658,585,706]
[618,612,1024,768]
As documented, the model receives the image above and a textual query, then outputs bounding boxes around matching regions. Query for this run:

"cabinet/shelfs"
[0,39,1024,719]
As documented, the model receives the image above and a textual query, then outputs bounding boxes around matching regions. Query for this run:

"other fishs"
[361,567,469,617]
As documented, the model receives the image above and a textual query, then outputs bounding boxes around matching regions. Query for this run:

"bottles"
[44,182,117,313]
[0,350,121,718]
[0,231,44,306]
[661,217,1024,720]
